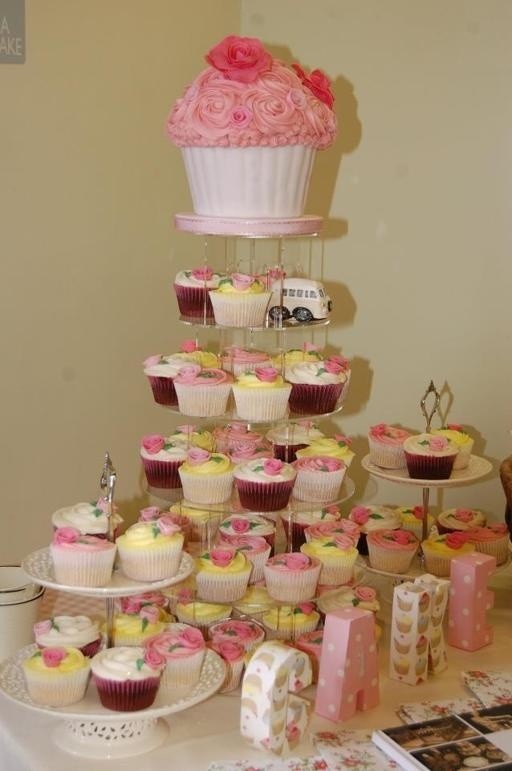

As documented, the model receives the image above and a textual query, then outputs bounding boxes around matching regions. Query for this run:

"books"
[372,703,512,771]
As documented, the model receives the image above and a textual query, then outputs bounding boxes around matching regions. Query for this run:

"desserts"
[24,340,511,712]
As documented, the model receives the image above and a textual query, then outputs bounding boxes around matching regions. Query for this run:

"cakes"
[165,33,339,218]
[172,265,286,327]
